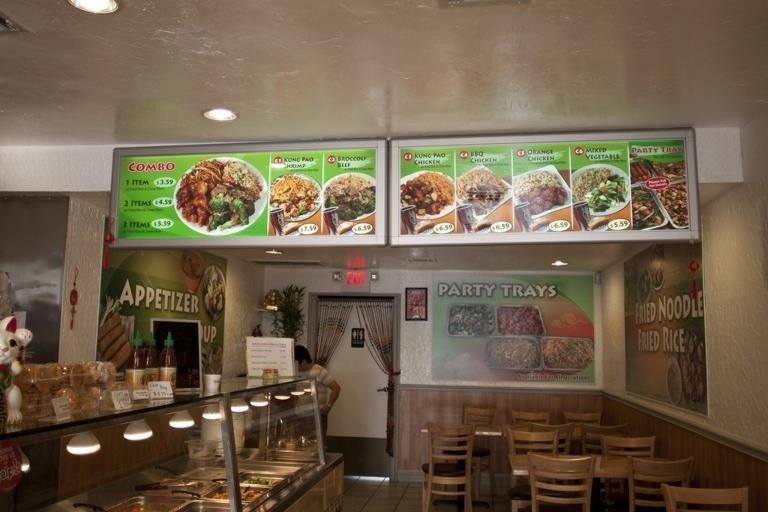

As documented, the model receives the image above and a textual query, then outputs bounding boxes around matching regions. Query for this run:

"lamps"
[66,433,101,457]
[122,419,153,441]
[200,386,310,422]
[169,411,195,429]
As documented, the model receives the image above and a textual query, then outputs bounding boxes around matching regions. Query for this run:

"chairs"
[598,434,659,506]
[525,419,576,455]
[562,410,604,422]
[625,453,697,511]
[419,421,478,510]
[504,427,561,503]
[525,451,595,511]
[440,406,500,499]
[659,480,749,511]
[510,410,552,424]
[578,421,630,457]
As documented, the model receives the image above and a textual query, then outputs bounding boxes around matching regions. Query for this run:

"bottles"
[124,327,178,395]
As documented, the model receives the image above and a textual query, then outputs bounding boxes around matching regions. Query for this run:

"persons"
[294,344,340,450]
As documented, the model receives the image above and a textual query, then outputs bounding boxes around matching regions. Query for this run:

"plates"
[322,171,376,221]
[636,268,667,305]
[172,156,268,237]
[664,359,683,406]
[631,180,689,231]
[201,263,227,320]
[270,172,323,223]
[399,165,630,221]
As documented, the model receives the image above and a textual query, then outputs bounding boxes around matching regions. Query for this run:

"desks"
[507,450,700,512]
[497,418,641,444]
[420,417,502,509]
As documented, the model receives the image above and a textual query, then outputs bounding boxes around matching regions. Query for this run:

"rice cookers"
[200,403,246,457]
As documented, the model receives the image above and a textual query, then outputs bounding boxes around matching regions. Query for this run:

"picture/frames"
[351,328,365,348]
[404,286,428,322]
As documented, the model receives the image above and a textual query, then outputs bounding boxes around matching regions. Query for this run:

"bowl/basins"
[186,440,217,460]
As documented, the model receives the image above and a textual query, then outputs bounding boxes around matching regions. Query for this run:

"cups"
[180,250,206,295]
[455,204,474,233]
[400,205,418,236]
[204,374,222,391]
[322,206,339,236]
[269,209,285,236]
[572,202,593,231]
[513,201,532,233]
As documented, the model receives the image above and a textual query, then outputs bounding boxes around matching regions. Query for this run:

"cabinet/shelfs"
[2,374,349,511]
